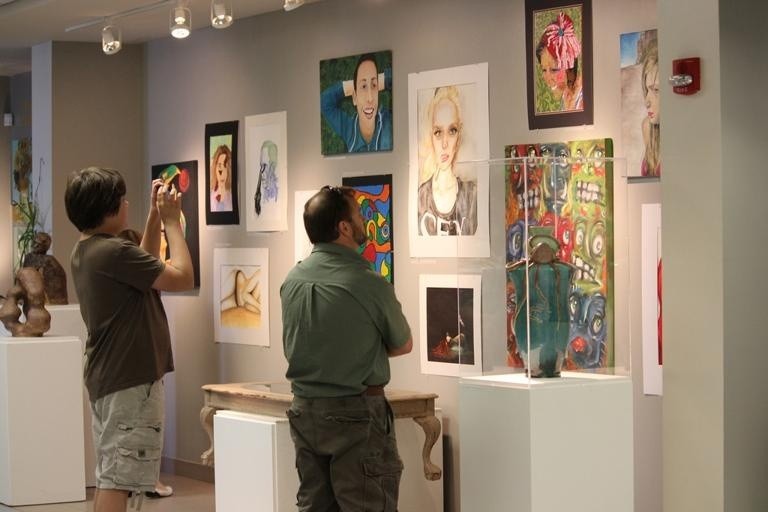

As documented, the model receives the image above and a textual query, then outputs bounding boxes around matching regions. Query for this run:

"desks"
[198,380,443,482]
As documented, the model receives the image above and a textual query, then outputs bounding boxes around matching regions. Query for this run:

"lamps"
[66,0,305,56]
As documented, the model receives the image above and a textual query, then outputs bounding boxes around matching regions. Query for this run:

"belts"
[366,386,384,395]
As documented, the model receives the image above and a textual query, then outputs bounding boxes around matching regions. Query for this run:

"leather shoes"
[145,486,171,497]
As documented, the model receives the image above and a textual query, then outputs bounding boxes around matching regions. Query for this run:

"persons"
[280,185,413,512]
[416,85,477,236]
[535,14,583,111]
[258,140,280,202]
[210,144,233,212]
[117,228,173,499]
[642,48,660,177]
[63,166,195,512]
[320,54,392,152]
